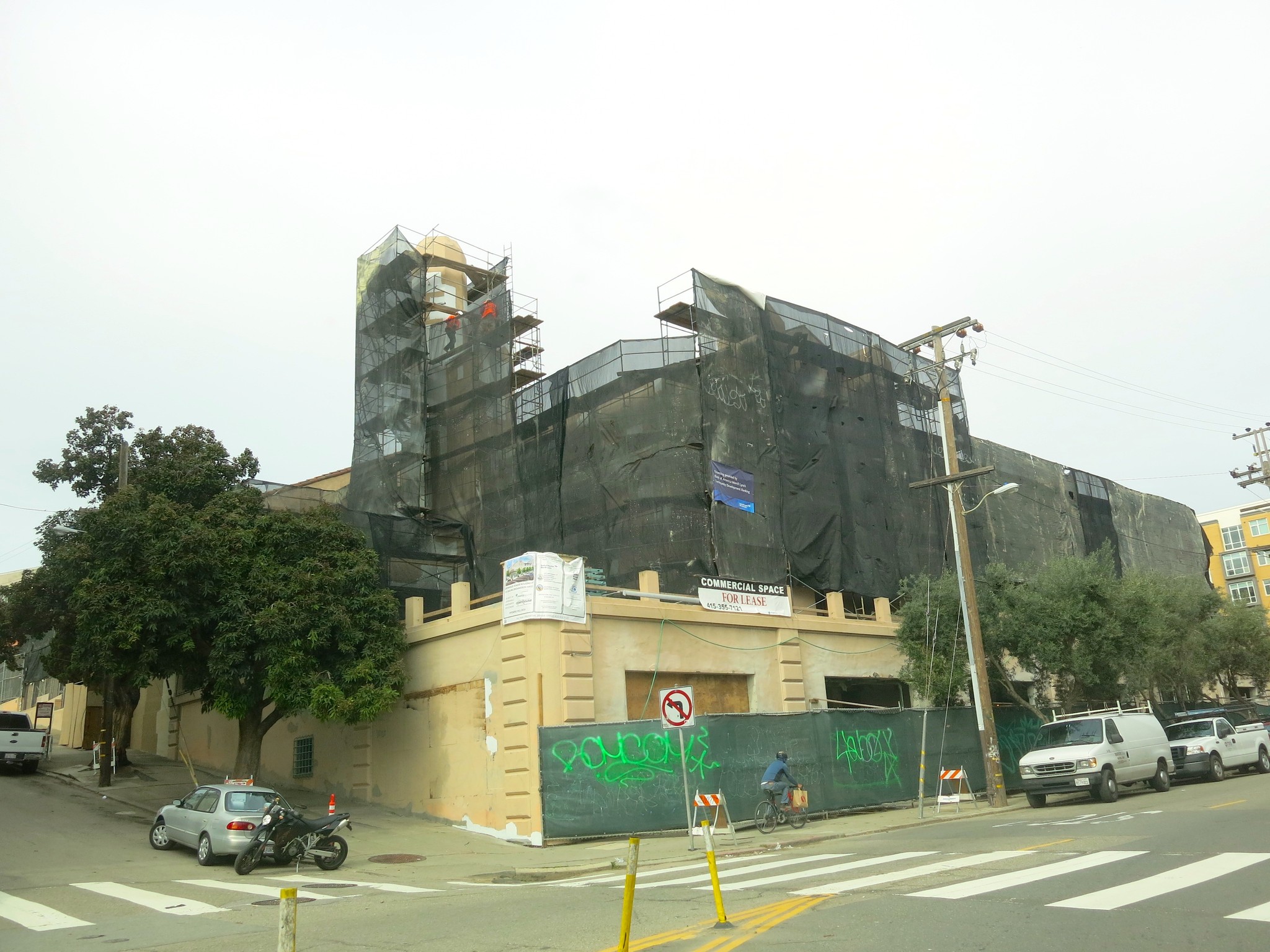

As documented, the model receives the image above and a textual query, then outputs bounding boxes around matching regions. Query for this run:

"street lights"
[948,481,1021,809]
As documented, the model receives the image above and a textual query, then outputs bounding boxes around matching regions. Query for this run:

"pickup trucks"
[1147,717,1270,789]
[0,710,48,774]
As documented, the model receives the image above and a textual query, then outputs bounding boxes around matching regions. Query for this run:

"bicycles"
[754,784,808,834]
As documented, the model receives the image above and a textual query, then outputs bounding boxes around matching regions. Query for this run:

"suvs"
[1019,711,1176,808]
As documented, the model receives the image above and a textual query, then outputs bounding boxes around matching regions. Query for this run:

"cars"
[149,784,298,866]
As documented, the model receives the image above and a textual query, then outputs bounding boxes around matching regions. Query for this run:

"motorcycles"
[234,785,353,876]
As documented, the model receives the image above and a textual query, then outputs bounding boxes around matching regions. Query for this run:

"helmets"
[777,750,788,759]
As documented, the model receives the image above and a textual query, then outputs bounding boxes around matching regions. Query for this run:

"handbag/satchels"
[793,787,809,808]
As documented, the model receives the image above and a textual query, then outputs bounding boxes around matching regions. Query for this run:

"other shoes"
[780,803,792,811]
[768,816,775,827]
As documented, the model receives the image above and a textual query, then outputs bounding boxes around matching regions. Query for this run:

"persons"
[761,751,802,817]
